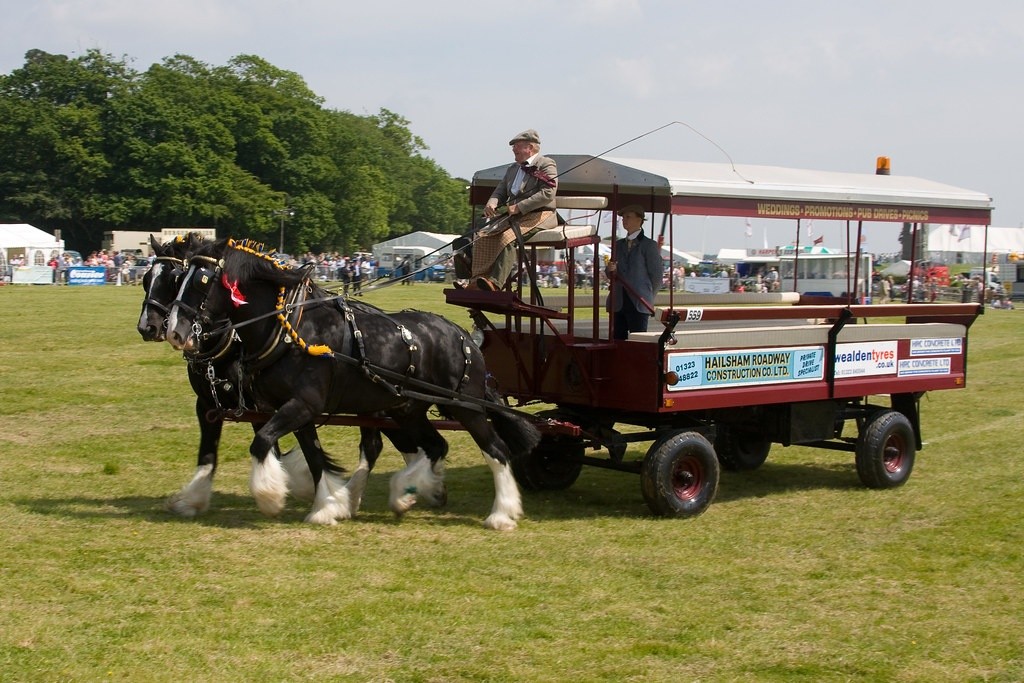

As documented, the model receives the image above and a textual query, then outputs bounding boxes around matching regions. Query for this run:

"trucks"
[377,245,446,284]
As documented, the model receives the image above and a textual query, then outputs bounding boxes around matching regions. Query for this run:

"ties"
[521,162,556,188]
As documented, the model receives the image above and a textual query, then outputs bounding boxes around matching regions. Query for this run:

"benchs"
[487,293,828,334]
[626,301,985,347]
[482,195,611,249]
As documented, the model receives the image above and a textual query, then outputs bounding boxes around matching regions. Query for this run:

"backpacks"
[50,260,56,266]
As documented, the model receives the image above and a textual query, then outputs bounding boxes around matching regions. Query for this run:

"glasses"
[512,143,529,149]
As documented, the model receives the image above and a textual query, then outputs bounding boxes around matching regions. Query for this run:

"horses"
[136,232,524,533]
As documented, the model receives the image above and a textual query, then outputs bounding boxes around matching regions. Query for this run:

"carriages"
[135,154,998,529]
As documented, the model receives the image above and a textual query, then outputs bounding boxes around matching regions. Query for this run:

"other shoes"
[477,277,500,292]
[453,281,486,293]
[354,293,357,296]
[359,295,363,296]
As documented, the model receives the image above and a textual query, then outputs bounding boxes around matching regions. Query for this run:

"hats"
[509,129,540,145]
[353,258,358,262]
[618,204,645,216]
[19,254,24,258]
[770,267,776,270]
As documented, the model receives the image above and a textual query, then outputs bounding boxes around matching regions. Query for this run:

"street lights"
[270,207,295,255]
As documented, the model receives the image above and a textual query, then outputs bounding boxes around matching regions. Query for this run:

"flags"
[948,224,958,237]
[744,218,752,237]
[808,219,817,236]
[957,224,971,243]
[813,237,823,245]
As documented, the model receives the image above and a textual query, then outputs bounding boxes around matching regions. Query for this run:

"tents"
[516,243,612,271]
[0,224,65,285]
[657,245,913,295]
[372,231,462,282]
[926,224,1024,299]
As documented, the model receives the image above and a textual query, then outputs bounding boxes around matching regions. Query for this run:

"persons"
[605,206,663,339]
[511,257,780,295]
[9,249,157,285]
[836,268,1015,311]
[280,252,454,296]
[453,130,558,293]
